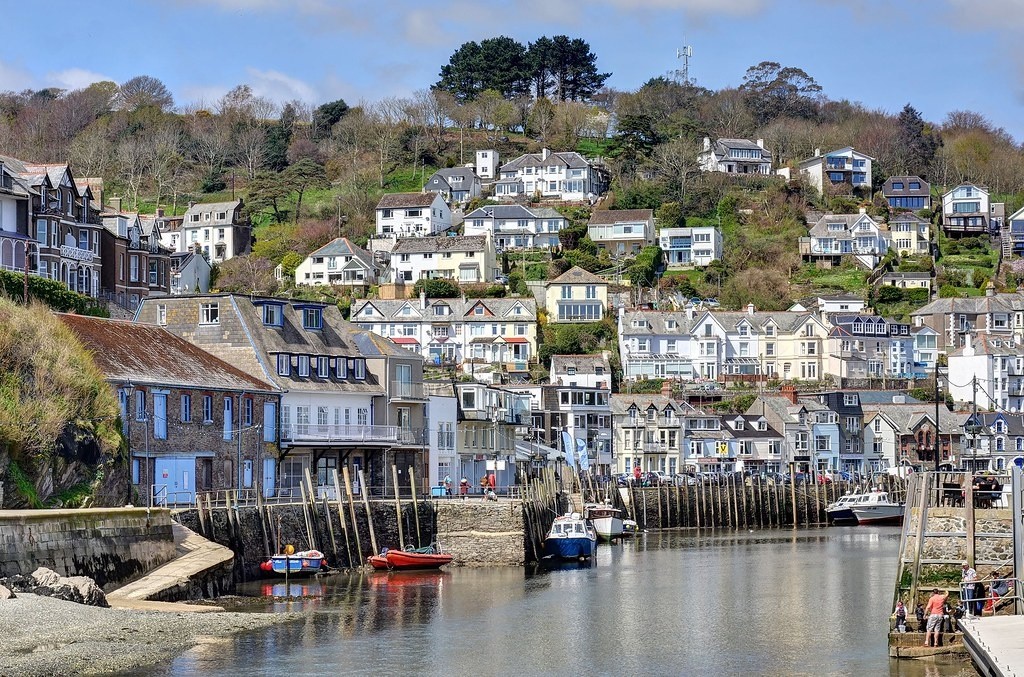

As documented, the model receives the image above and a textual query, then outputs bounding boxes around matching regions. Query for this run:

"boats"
[585,502,624,541]
[270,550,325,574]
[823,486,899,526]
[367,554,394,570]
[622,518,638,536]
[543,512,599,561]
[850,503,907,526]
[387,549,454,568]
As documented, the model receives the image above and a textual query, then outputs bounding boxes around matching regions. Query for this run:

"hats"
[917,603,924,606]
[962,562,968,566]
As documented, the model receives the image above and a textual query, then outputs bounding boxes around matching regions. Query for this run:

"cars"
[580,464,914,492]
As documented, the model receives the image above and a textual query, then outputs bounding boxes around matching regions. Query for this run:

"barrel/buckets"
[899,624,907,633]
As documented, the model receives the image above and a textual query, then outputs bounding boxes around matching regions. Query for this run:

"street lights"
[255,421,264,505]
[122,379,135,508]
[420,426,430,501]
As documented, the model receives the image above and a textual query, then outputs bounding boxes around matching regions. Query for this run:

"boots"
[993,592,1003,604]
[985,599,992,611]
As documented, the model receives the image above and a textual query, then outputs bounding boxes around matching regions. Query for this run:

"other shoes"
[924,641,929,646]
[934,643,939,647]
[918,630,924,633]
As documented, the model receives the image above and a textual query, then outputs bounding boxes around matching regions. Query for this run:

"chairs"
[940,483,1003,509]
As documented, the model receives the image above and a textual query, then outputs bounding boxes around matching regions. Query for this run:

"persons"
[915,603,927,633]
[961,473,1000,509]
[961,562,978,614]
[943,603,955,633]
[480,473,498,502]
[444,474,452,499]
[953,600,965,619]
[459,477,471,500]
[923,589,949,647]
[891,602,908,628]
[528,471,536,484]
[634,464,641,487]
[983,571,1009,611]
